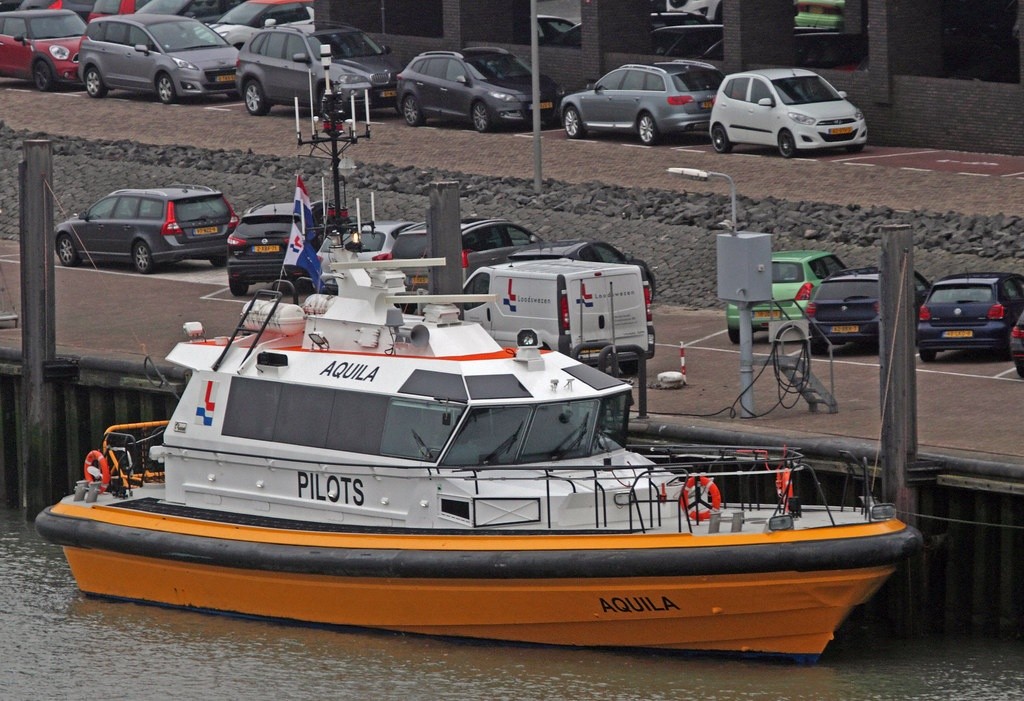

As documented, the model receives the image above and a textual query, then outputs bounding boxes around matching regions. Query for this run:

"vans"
[134,0,247,23]
[77,13,240,105]
[702,25,846,63]
[461,258,655,374]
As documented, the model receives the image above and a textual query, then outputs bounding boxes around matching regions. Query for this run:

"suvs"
[506,238,655,301]
[391,216,576,316]
[53,182,240,275]
[559,59,725,146]
[227,199,353,296]
[236,19,400,119]
[559,10,711,51]
[313,219,417,295]
[395,44,564,133]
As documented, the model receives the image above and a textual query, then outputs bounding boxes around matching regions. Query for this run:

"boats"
[34,41,923,665]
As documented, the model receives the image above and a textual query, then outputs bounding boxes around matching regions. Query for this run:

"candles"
[356,197,361,234]
[351,95,355,137]
[371,191,375,221]
[365,89,370,131]
[295,96,300,138]
[322,176,325,216]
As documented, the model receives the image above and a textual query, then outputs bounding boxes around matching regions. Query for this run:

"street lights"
[666,168,755,418]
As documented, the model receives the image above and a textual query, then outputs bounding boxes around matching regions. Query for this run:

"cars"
[726,248,850,344]
[0,9,88,92]
[537,14,576,48]
[805,266,931,354]
[88,0,151,22]
[919,271,1024,381]
[650,23,725,59]
[206,0,315,50]
[15,0,94,20]
[710,66,868,157]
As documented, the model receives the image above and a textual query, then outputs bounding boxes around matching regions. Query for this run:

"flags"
[293,176,316,240]
[283,222,323,296]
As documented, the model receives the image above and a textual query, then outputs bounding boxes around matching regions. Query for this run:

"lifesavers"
[680,476,721,521]
[775,465,793,512]
[84,450,111,494]
